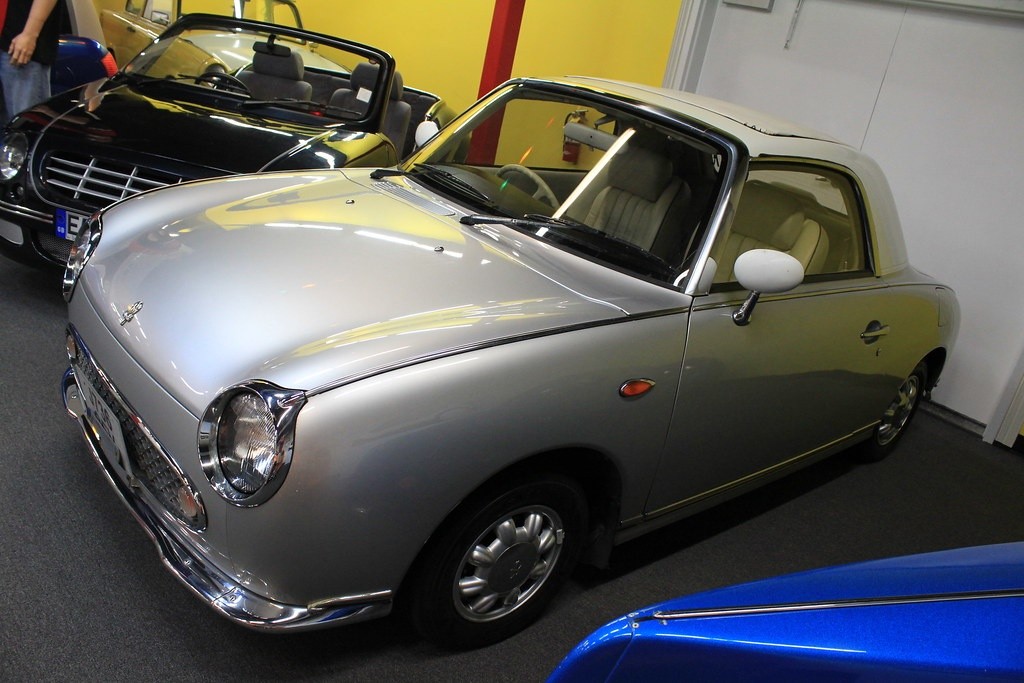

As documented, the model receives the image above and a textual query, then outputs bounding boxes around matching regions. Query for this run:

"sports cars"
[70,76,960,648]
[0,14,476,272]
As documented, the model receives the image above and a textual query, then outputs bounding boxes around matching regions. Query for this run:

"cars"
[100,0,352,90]
[543,537,1024,683]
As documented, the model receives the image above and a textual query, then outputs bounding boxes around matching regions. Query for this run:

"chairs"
[237,51,312,107]
[712,180,830,284]
[584,147,694,266]
[328,62,411,160]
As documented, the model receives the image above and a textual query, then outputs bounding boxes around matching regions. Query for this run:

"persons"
[0,0,62,122]
